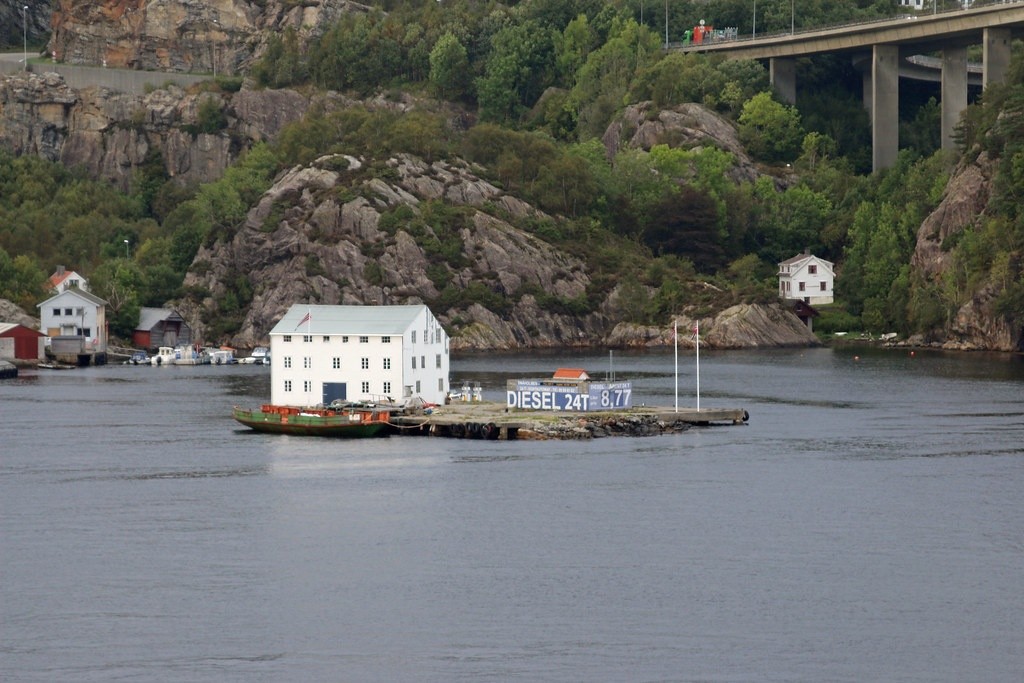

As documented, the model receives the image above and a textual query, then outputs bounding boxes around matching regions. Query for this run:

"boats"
[123,345,271,367]
[221,403,389,438]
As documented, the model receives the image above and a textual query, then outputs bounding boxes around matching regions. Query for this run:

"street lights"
[123,238,131,257]
[80,313,90,355]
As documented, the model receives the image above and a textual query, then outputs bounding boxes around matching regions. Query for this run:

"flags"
[669,327,675,341]
[692,325,698,333]
